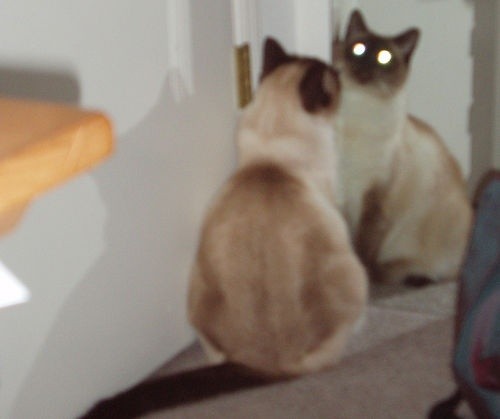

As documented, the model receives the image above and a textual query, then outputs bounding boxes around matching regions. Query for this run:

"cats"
[336,7,473,285]
[188,36,369,378]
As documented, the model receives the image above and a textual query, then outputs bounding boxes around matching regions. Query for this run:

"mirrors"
[247,0,500,320]
